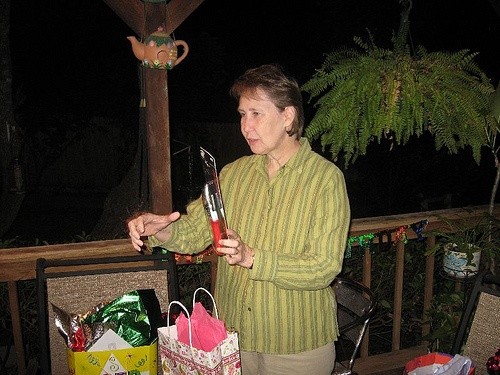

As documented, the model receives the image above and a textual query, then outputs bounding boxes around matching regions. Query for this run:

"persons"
[128,62,351,375]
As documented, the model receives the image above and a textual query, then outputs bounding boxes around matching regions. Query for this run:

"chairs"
[36,252,180,375]
[330,275,500,375]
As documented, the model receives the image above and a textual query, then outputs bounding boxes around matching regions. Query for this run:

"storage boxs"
[66,342,157,375]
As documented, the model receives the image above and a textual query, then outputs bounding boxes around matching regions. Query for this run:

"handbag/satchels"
[66,341,158,375]
[158,287,241,375]
[404,352,476,375]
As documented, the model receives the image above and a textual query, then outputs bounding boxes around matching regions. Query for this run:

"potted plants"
[425,206,500,279]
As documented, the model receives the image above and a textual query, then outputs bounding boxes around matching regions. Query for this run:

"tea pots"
[127,26,189,70]
